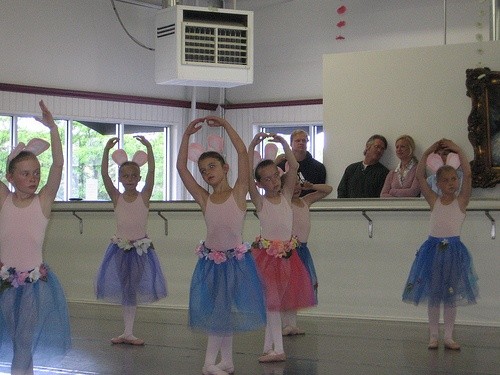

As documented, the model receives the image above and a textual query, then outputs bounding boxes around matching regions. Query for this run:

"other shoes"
[258,353,287,362]
[428,340,438,349]
[290,328,306,335]
[111,336,124,344]
[443,339,461,350]
[216,362,235,373]
[202,366,229,375]
[122,334,144,345]
[282,327,292,335]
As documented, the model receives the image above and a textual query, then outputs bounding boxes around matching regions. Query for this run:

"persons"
[273,129,327,198]
[176,116,267,375]
[95,135,168,346]
[420,145,464,198]
[247,132,318,362]
[276,161,333,336]
[380,135,427,198]
[337,134,390,198]
[402,139,481,350]
[0,99,72,375]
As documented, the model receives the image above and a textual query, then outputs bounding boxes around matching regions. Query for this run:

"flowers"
[194,235,305,264]
[0,259,49,289]
[110,232,153,256]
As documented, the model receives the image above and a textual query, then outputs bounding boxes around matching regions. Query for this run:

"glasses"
[371,145,386,151]
